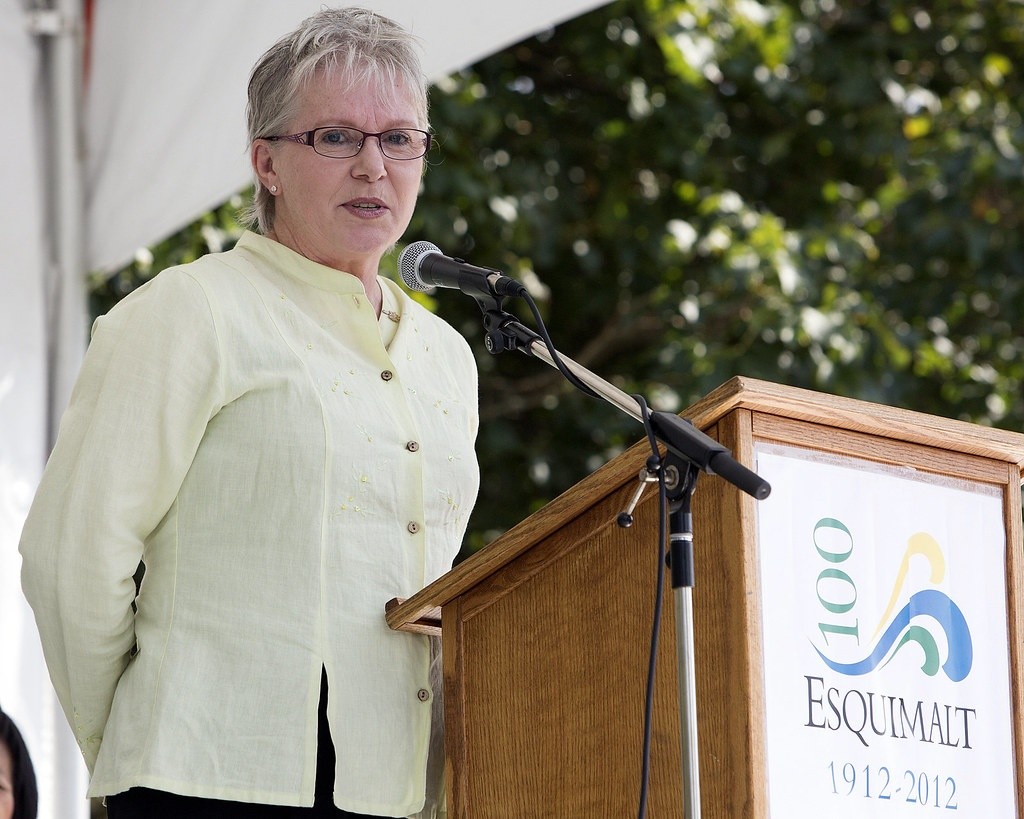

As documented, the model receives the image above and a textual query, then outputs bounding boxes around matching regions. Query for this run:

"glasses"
[251,126,432,159]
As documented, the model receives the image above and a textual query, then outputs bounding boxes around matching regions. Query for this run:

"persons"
[0,711,42,819]
[18,7,485,819]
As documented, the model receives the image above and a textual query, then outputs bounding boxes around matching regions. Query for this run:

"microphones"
[397,241,525,296]
